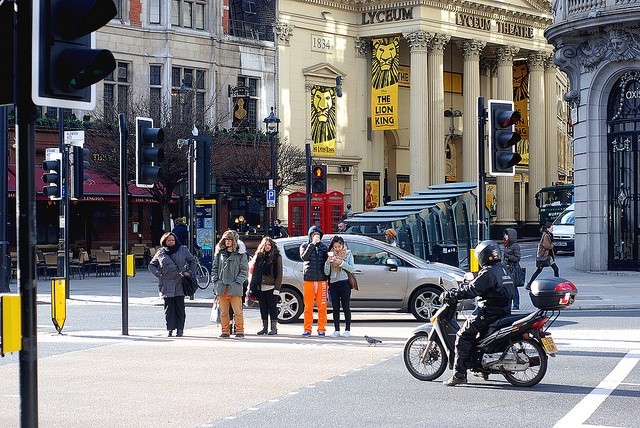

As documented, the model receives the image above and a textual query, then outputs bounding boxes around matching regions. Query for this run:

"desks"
[263,106,281,238]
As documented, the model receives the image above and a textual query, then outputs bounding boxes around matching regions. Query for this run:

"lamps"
[36,250,47,280]
[81,251,96,274]
[69,252,84,279]
[131,246,148,270]
[106,250,118,260]
[150,248,156,257]
[70,247,87,275]
[90,249,102,264]
[9,251,17,283]
[42,251,57,255]
[95,252,114,276]
[45,255,58,275]
[134,243,146,247]
[99,245,114,250]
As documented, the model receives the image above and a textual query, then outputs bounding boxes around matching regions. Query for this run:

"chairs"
[217,333,230,339]
[443,375,467,386]
[343,332,350,337]
[469,368,488,380]
[302,332,311,337]
[235,334,244,340]
[318,332,325,338]
[330,331,340,338]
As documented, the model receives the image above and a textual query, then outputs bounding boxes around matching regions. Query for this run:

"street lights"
[536,256,549,267]
[348,272,358,290]
[507,268,526,287]
[210,296,221,324]
[181,276,198,296]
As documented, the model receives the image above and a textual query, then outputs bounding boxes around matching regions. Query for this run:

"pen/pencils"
[550,203,574,254]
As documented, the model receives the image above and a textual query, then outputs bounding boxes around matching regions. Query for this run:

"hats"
[242,234,476,324]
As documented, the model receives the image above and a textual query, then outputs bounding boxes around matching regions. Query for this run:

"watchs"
[403,272,578,386]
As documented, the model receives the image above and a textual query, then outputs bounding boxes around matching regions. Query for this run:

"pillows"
[474,240,502,268]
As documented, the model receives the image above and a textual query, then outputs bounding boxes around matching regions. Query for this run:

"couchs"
[43,159,60,197]
[135,117,164,189]
[68,146,90,200]
[488,99,522,177]
[312,165,327,192]
[32,0,117,110]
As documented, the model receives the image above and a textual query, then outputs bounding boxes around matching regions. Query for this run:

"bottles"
[219,230,239,252]
[387,230,396,237]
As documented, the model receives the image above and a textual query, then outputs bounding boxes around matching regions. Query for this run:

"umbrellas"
[168,330,172,337]
[176,330,182,337]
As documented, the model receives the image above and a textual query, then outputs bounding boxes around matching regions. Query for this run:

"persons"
[335,204,361,234]
[226,216,289,238]
[443,240,514,386]
[211,230,248,339]
[384,229,399,248]
[148,232,196,337]
[324,236,354,338]
[249,236,283,335]
[172,217,188,246]
[501,228,521,310]
[229,280,248,336]
[525,222,559,290]
[300,225,330,337]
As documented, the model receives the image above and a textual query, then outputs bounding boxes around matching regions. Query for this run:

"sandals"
[535,182,574,237]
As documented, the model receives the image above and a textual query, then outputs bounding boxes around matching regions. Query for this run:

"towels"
[434,244,459,268]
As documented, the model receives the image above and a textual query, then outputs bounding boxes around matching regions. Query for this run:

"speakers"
[257,320,268,335]
[269,320,277,335]
